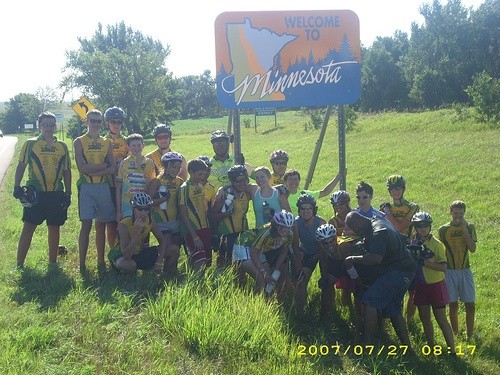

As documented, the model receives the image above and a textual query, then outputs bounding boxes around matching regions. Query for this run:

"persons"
[105,194,168,271]
[231,209,294,300]
[343,209,419,350]
[12,110,72,272]
[407,211,457,356]
[293,194,334,316]
[178,159,212,277]
[71,106,477,356]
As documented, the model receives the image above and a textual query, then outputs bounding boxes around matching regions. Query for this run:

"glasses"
[415,223,430,228]
[262,201,275,216]
[89,119,103,124]
[323,238,335,244]
[301,207,313,211]
[136,206,149,211]
[355,195,371,199]
[111,121,123,125]
[387,188,403,190]
[276,161,286,166]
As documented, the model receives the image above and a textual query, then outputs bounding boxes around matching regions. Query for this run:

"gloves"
[61,191,73,212]
[12,185,24,199]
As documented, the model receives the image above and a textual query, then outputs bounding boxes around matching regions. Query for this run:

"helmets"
[152,124,248,182]
[270,151,289,163]
[411,211,433,224]
[272,209,294,228]
[20,186,43,208]
[329,190,350,205]
[296,194,318,216]
[130,193,153,208]
[103,107,126,121]
[387,175,405,187]
[314,223,338,242]
